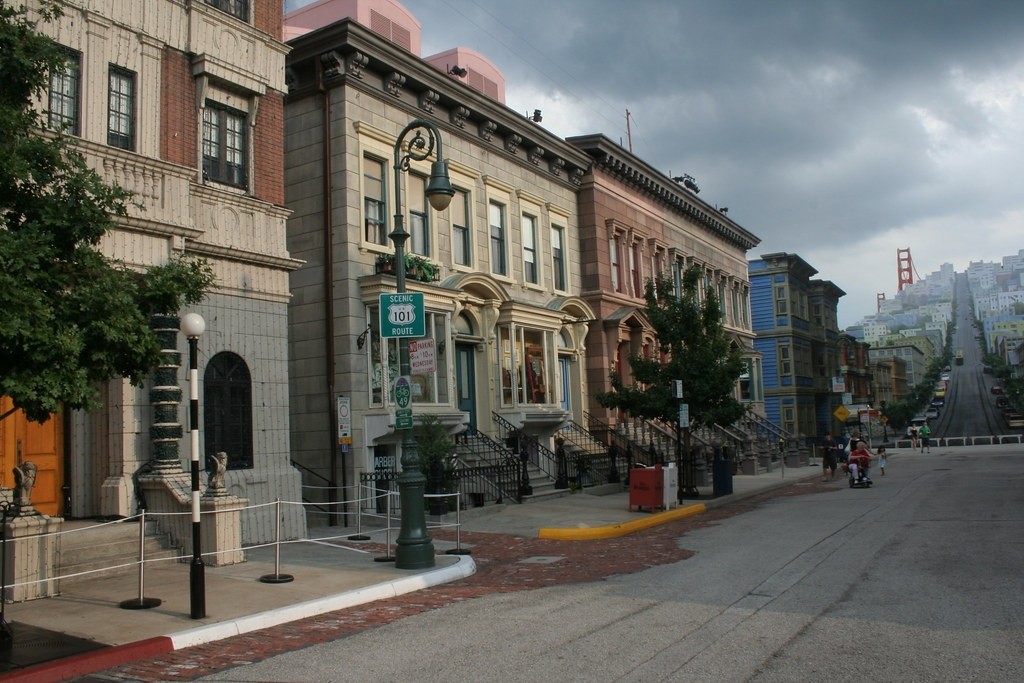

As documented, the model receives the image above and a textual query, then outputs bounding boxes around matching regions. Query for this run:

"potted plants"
[378,251,440,282]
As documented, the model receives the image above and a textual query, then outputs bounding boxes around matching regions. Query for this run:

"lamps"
[529,109,543,123]
[447,64,467,78]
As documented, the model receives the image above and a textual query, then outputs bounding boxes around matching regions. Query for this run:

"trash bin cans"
[643,462,679,512]
[628,463,664,514]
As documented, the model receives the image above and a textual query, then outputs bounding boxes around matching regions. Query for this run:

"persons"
[821,422,931,482]
[518,355,536,403]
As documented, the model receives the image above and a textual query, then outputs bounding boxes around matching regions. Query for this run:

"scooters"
[848,456,874,488]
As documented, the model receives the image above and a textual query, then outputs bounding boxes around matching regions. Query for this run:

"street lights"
[181,313,207,621]
[388,117,455,572]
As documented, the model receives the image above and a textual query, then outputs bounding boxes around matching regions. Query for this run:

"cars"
[943,364,952,372]
[996,396,1024,429]
[907,373,949,437]
[991,386,1002,394]
[982,366,993,373]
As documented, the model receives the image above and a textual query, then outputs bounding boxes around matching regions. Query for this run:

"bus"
[956,350,964,365]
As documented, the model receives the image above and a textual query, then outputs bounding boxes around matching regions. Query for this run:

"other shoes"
[863,477,868,481]
[854,480,858,484]
[832,477,836,480]
[822,476,827,481]
